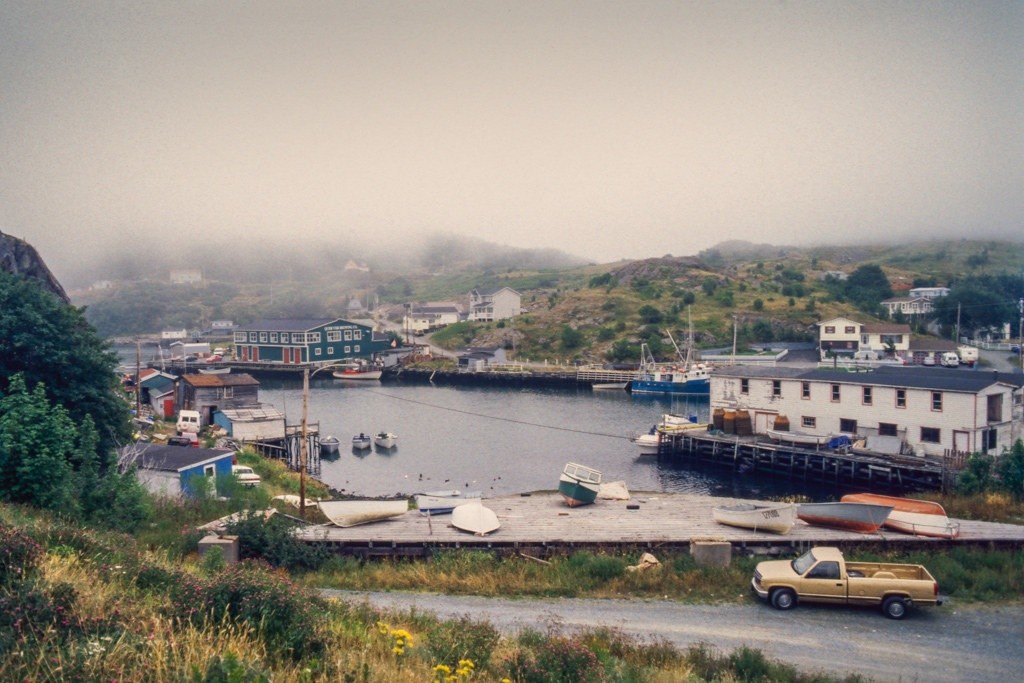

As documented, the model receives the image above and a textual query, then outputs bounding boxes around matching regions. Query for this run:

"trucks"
[956,345,979,367]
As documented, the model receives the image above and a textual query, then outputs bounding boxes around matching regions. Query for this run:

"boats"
[450,502,502,537]
[332,367,382,380]
[657,423,708,437]
[196,366,231,375]
[624,304,710,394]
[795,501,895,534]
[413,492,482,515]
[596,480,631,501]
[839,491,962,540]
[632,390,700,455]
[319,435,340,454]
[351,433,372,449]
[558,462,603,509]
[712,501,795,535]
[590,379,629,390]
[373,431,399,449]
[317,497,410,528]
[765,428,832,445]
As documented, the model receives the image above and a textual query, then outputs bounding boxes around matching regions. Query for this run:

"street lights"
[730,316,737,365]
[134,339,160,418]
[299,363,361,524]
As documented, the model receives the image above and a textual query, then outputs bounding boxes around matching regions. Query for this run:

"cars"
[270,493,318,511]
[208,447,238,465]
[166,435,193,449]
[170,347,226,363]
[180,430,200,448]
[231,463,262,490]
[922,357,936,366]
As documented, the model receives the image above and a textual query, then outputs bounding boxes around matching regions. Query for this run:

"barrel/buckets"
[689,416,697,424]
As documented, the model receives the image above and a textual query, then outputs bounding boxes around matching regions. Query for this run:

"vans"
[940,352,960,368]
[175,409,201,436]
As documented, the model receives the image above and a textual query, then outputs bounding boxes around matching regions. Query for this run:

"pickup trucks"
[750,546,944,621]
